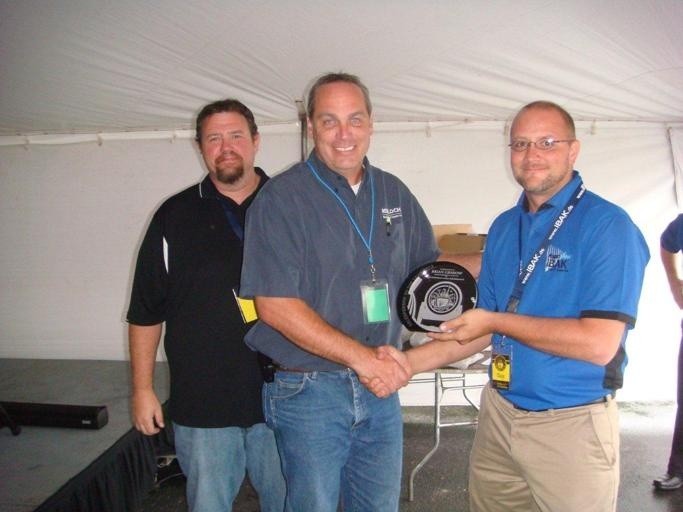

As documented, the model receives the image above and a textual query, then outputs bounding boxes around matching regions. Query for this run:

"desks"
[399,341,494,504]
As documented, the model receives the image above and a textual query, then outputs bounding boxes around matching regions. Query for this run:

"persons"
[651,211,682,492]
[238,71,484,511]
[359,99,650,511]
[125,98,287,512]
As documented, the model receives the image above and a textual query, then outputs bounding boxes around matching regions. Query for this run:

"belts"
[268,361,345,373]
[510,391,616,414]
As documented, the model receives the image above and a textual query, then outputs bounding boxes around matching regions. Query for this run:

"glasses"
[508,136,576,150]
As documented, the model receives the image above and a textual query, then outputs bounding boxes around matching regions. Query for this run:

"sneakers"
[651,470,682,496]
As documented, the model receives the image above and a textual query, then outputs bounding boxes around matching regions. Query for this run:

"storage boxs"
[425,223,488,253]
